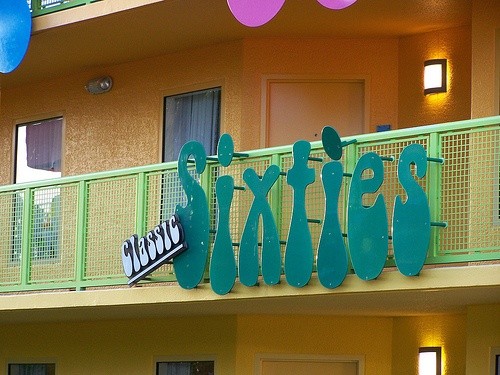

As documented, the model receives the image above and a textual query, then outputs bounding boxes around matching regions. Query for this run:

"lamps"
[84,74,113,94]
[422,59,448,96]
[418,346,441,375]
